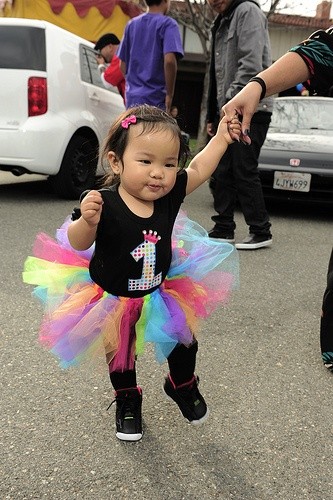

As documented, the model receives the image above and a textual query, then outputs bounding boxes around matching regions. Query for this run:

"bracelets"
[247,77,267,100]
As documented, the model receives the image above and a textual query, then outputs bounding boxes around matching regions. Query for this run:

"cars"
[255,96,333,214]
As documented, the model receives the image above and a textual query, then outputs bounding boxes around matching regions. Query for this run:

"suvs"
[0,17,125,199]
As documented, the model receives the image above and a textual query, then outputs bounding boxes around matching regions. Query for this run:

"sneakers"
[207,231,234,243]
[162,373,208,426]
[105,386,143,440]
[234,232,272,249]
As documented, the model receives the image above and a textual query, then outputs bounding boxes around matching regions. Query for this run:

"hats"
[93,33,119,50]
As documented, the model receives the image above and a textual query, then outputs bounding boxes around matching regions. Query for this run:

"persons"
[115,0,185,117]
[94,33,128,105]
[204,0,274,249]
[222,26,333,376]
[23,105,242,440]
